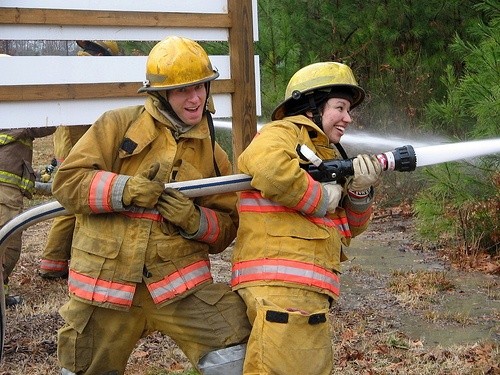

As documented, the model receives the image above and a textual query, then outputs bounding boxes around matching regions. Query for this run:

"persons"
[233,62,382,375]
[51,36,252,375]
[0,126,58,309]
[40,40,120,278]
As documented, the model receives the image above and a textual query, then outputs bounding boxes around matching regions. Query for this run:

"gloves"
[121,162,161,209]
[321,180,343,214]
[351,153,382,191]
[156,187,200,234]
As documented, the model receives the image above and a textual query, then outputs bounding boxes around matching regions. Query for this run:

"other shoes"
[5,296,17,308]
[39,269,68,280]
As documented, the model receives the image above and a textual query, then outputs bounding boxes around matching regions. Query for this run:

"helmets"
[136,35,219,93]
[75,39,118,56]
[271,62,366,122]
